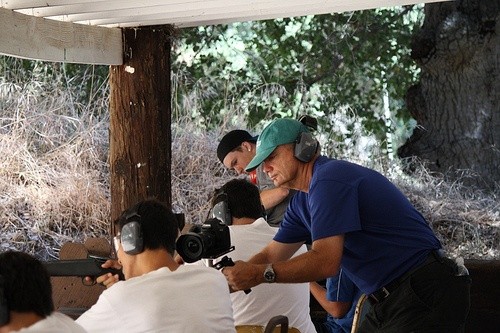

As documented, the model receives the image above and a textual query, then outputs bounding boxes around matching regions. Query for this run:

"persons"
[0,251,87,333]
[221,117,471,333]
[212,178,318,333]
[217,129,371,333]
[73,199,237,333]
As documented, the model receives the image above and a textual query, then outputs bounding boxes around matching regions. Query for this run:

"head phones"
[120,202,145,255]
[294,115,319,163]
[212,180,233,226]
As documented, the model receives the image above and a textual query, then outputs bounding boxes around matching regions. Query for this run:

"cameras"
[175,218,230,263]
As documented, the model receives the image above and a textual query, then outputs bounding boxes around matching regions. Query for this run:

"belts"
[365,249,446,306]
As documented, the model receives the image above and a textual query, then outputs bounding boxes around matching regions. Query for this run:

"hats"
[217,129,259,163]
[245,117,310,172]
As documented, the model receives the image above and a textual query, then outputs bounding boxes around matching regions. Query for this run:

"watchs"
[264,264,275,283]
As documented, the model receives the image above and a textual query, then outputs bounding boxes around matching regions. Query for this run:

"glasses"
[114,234,122,252]
